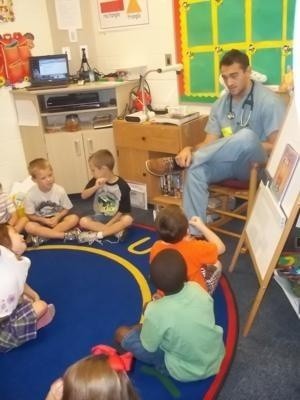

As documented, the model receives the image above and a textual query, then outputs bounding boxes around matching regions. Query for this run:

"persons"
[149,204,226,299]
[114,248,226,383]
[79,149,134,245]
[45,344,140,400]
[144,49,285,240]
[0,158,82,355]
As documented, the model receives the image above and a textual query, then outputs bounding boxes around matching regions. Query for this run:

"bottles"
[65,114,79,129]
[80,48,91,82]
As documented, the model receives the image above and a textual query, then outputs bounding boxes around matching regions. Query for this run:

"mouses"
[77,79,85,86]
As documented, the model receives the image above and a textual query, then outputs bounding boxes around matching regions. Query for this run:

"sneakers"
[77,231,103,246]
[36,303,56,331]
[65,228,81,242]
[31,234,45,247]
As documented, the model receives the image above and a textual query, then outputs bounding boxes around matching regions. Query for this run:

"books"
[157,171,184,198]
[270,143,300,206]
[149,111,199,126]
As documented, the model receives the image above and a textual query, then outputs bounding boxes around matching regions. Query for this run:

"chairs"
[208,92,290,254]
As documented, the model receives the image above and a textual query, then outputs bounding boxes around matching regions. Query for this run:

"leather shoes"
[144,156,185,178]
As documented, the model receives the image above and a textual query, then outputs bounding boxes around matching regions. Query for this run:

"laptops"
[25,54,70,91]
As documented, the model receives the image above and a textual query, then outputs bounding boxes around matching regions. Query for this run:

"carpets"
[0,223,239,400]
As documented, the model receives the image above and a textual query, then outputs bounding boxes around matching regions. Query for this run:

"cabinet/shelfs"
[9,76,142,196]
[112,114,208,210]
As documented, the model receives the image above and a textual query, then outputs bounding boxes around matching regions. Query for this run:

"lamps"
[125,63,185,123]
